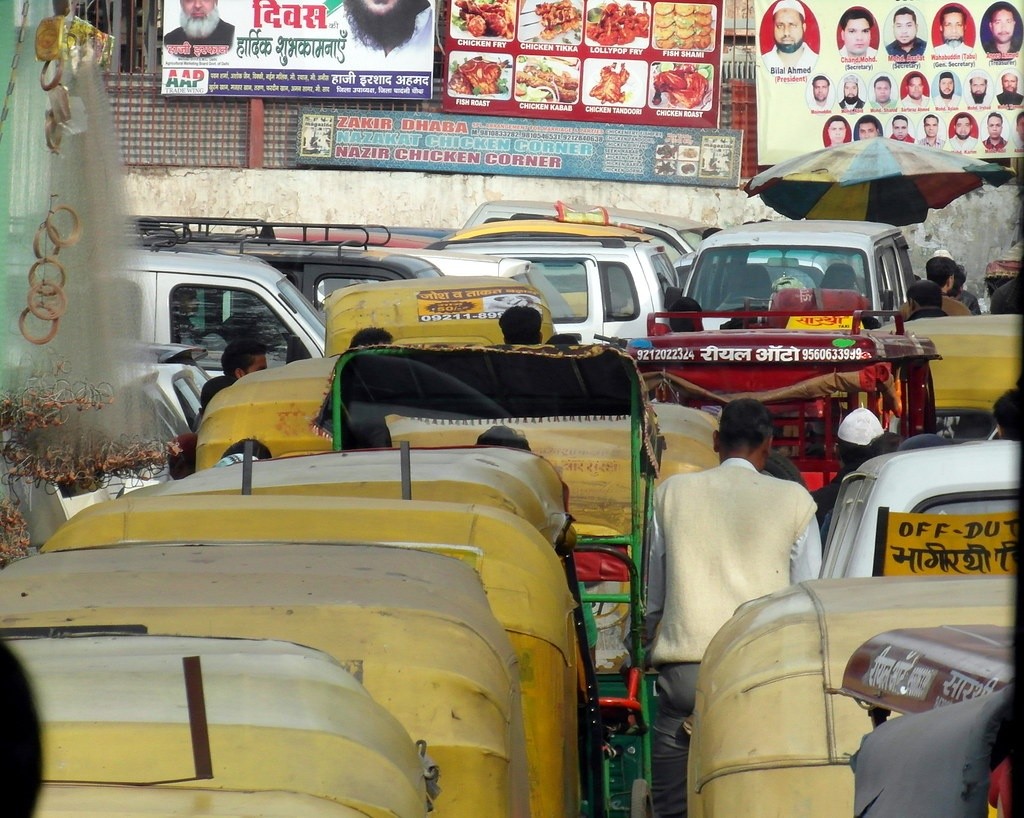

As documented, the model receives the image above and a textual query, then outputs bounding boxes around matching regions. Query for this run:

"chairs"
[821,263,859,291]
[731,283,735,298]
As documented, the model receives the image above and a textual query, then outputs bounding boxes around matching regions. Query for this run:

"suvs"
[8,205,328,374]
[115,211,446,298]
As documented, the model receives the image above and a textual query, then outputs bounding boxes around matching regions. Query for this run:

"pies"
[654,3,712,49]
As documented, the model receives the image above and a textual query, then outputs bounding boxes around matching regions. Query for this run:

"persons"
[123,341,272,494]
[642,377,1024,818]
[163,0,236,57]
[724,263,771,305]
[499,305,542,344]
[899,249,1024,322]
[761,0,1024,153]
[818,263,856,289]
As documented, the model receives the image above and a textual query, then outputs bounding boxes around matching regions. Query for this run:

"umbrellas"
[745,137,1017,228]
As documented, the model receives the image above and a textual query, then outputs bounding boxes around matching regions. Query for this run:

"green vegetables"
[450,57,509,97]
[516,55,564,101]
[450,0,496,31]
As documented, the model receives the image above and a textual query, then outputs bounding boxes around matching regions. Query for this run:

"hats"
[477,426,531,450]
[929,250,955,261]
[969,71,988,81]
[837,408,884,445]
[773,0,806,20]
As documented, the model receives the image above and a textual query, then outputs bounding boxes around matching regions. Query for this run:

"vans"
[259,196,914,326]
[819,438,1024,585]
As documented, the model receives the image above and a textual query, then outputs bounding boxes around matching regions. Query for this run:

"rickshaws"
[3,273,1024,817]
